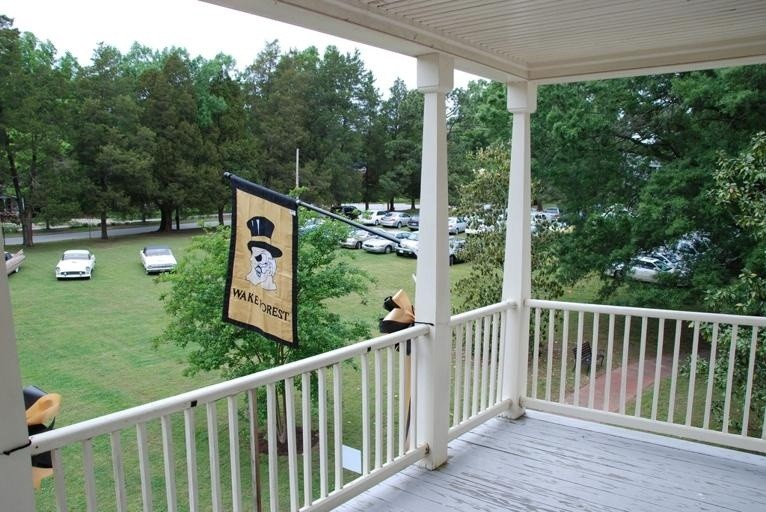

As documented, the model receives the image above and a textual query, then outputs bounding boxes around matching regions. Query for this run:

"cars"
[597,232,712,287]
[301,203,570,269]
[54,249,98,281]
[610,204,628,216]
[4,246,27,278]
[138,243,178,275]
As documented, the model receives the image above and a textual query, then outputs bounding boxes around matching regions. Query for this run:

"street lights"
[378,288,414,453]
[22,383,61,491]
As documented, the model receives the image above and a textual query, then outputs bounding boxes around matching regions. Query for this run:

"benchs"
[572,341,604,375]
